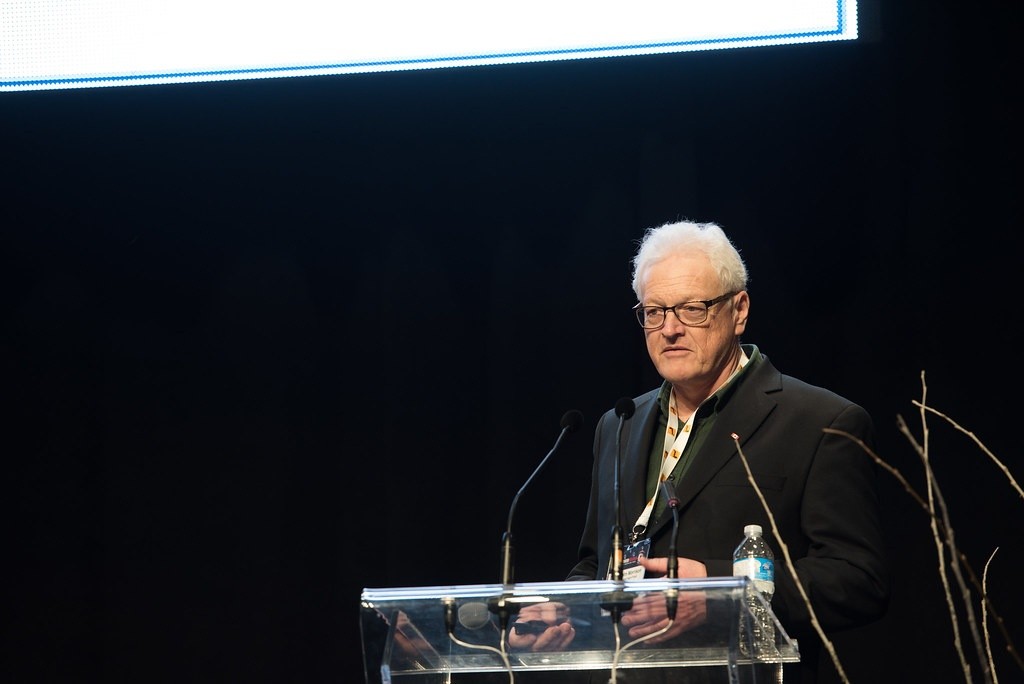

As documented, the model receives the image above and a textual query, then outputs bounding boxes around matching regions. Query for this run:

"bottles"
[731,524,776,657]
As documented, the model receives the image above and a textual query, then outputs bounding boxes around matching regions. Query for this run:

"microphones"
[611,398,635,579]
[662,480,680,580]
[501,410,585,585]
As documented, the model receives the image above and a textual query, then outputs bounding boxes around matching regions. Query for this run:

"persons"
[506,219,888,684]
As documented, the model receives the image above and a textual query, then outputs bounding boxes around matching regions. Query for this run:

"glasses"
[633,291,738,329]
